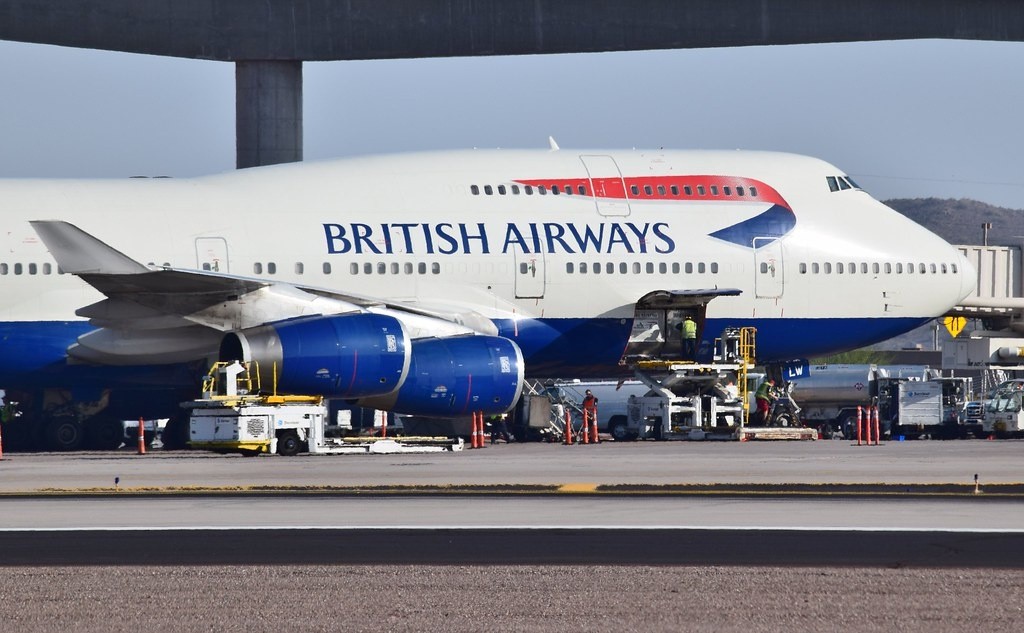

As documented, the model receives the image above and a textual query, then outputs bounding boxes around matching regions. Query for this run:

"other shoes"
[506,439,510,444]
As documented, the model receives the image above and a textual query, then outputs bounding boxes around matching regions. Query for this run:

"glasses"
[586,394,590,395]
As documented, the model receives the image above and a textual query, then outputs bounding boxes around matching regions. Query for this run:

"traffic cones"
[479,413,486,446]
[135,417,146,455]
[581,405,590,443]
[593,409,599,443]
[564,406,573,445]
[469,413,477,448]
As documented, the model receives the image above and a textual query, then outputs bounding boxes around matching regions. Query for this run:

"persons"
[484,414,512,444]
[675,314,697,361]
[0,396,15,422]
[752,380,778,425]
[582,390,598,432]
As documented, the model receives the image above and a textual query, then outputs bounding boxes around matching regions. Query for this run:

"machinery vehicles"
[615,287,757,440]
[180,360,462,457]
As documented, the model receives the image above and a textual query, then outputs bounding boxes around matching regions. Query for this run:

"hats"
[2,397,9,400]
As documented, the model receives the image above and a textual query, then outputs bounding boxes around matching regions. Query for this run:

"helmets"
[686,313,691,319]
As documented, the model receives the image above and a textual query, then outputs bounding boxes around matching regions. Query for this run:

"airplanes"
[0,150,978,450]
